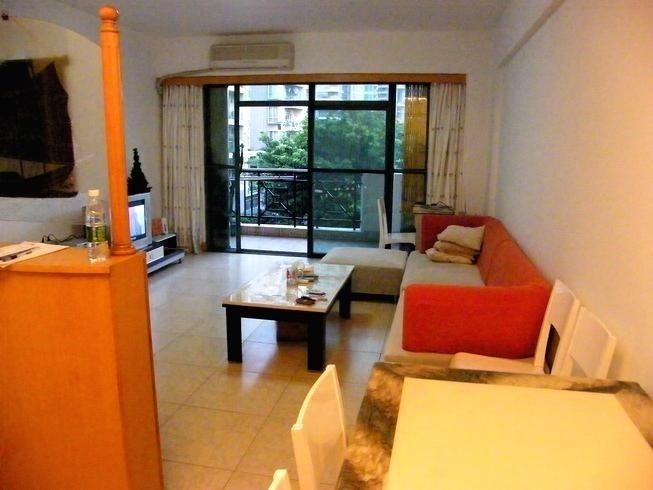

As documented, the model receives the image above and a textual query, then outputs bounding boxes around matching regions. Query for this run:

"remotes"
[308,292,326,296]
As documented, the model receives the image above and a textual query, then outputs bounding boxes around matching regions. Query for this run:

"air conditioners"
[209,42,295,71]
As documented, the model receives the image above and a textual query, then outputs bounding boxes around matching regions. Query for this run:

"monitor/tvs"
[127,192,153,252]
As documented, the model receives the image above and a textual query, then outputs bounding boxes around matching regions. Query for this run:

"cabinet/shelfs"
[138,233,185,274]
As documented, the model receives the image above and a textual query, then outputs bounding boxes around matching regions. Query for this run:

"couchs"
[383,213,552,364]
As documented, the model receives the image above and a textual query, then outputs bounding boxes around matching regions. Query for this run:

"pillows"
[425,225,486,264]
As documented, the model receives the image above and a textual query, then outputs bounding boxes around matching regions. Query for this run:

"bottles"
[85,189,108,263]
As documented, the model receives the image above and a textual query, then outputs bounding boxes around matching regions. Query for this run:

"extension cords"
[38,240,58,245]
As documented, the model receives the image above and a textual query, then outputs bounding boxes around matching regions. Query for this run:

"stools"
[319,246,409,306]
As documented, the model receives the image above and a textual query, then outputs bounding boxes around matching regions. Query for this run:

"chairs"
[450,278,581,376]
[557,306,617,380]
[269,469,292,490]
[377,196,416,250]
[290,363,348,490]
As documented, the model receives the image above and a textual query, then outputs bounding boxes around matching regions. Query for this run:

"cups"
[286,268,303,285]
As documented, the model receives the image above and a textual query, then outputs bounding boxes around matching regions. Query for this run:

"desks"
[412,204,454,250]
[332,362,652,490]
